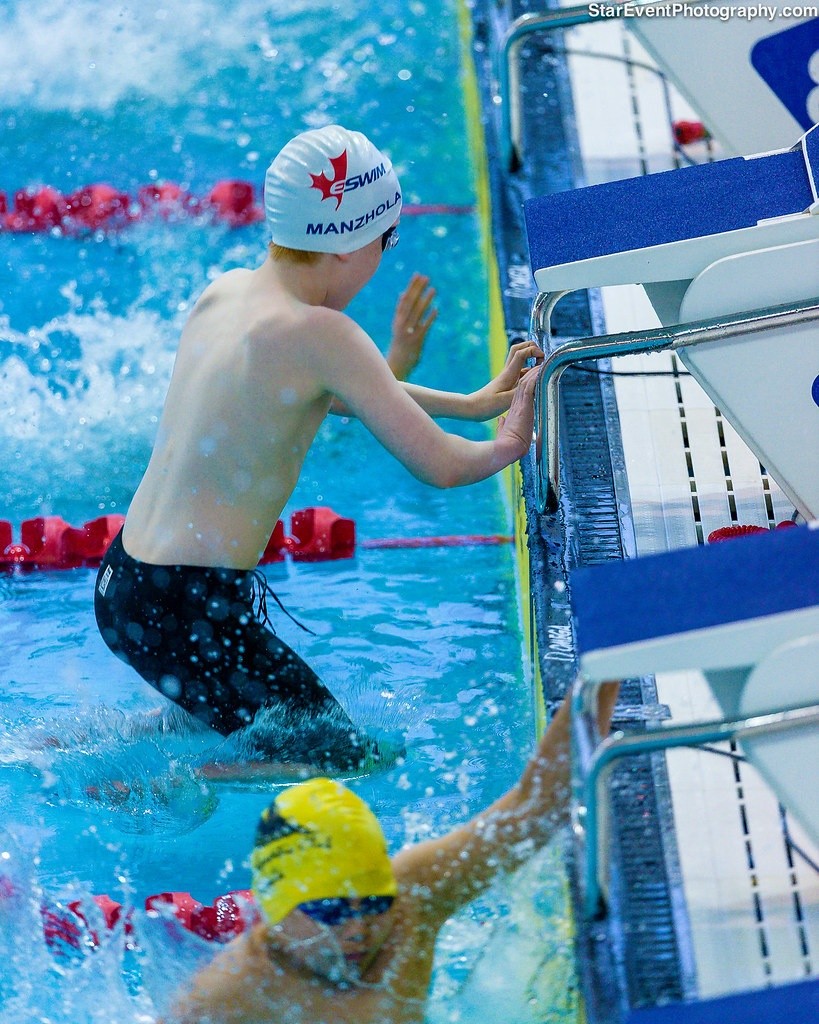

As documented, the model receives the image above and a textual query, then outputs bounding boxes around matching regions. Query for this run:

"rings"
[405,325,416,334]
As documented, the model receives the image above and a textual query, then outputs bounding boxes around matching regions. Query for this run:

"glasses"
[383,229,400,248]
[294,894,393,924]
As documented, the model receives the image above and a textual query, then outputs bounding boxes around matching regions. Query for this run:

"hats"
[264,124,403,254]
[247,778,400,925]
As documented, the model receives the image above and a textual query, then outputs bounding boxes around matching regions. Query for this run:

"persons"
[147,671,621,1024]
[92,124,546,789]
